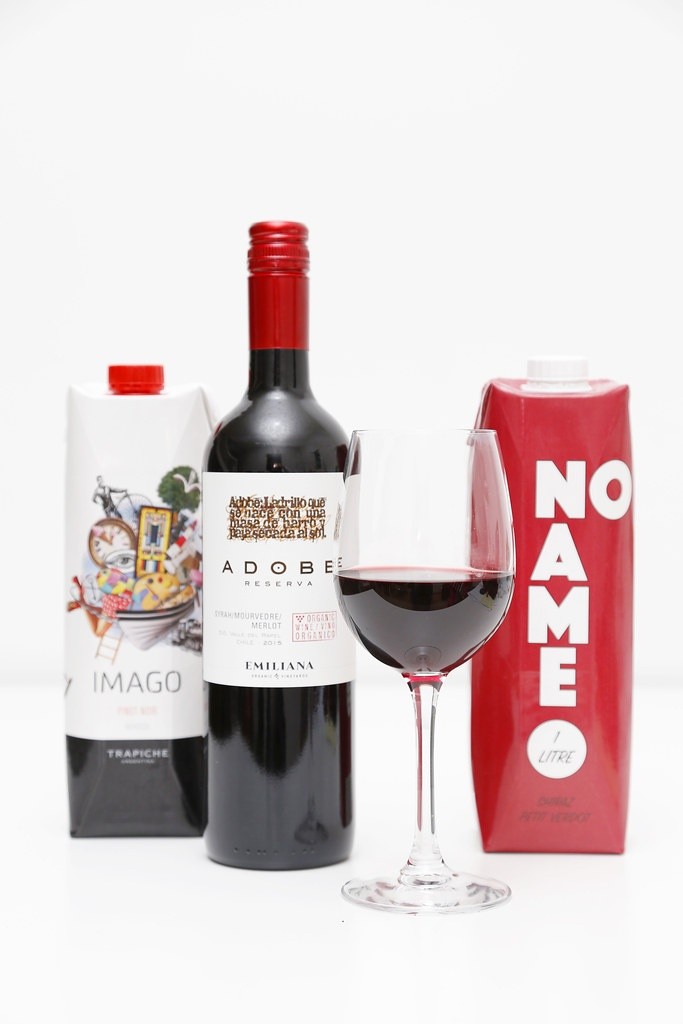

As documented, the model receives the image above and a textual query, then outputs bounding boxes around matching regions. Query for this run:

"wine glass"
[332,426,517,911]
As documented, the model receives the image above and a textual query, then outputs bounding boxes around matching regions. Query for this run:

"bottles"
[66,363,208,838]
[200,222,361,873]
